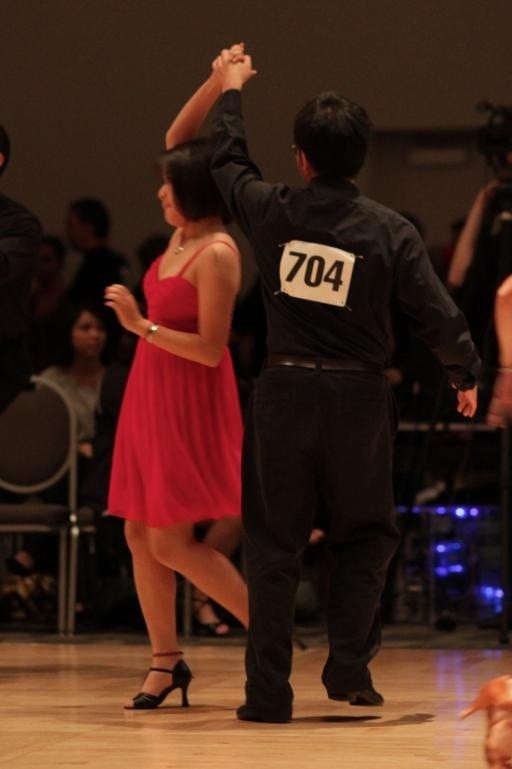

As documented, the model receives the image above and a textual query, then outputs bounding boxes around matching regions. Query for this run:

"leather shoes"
[236,705,294,723]
[327,681,384,707]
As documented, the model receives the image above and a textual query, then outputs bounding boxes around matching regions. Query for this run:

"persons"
[1,125,512,641]
[99,38,307,713]
[210,42,487,725]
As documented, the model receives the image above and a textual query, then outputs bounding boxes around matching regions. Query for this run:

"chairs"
[0,377,97,636]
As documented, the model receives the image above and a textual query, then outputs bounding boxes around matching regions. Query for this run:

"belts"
[265,351,359,373]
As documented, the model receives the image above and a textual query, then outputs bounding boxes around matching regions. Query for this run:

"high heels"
[459,675,512,767]
[123,658,193,708]
